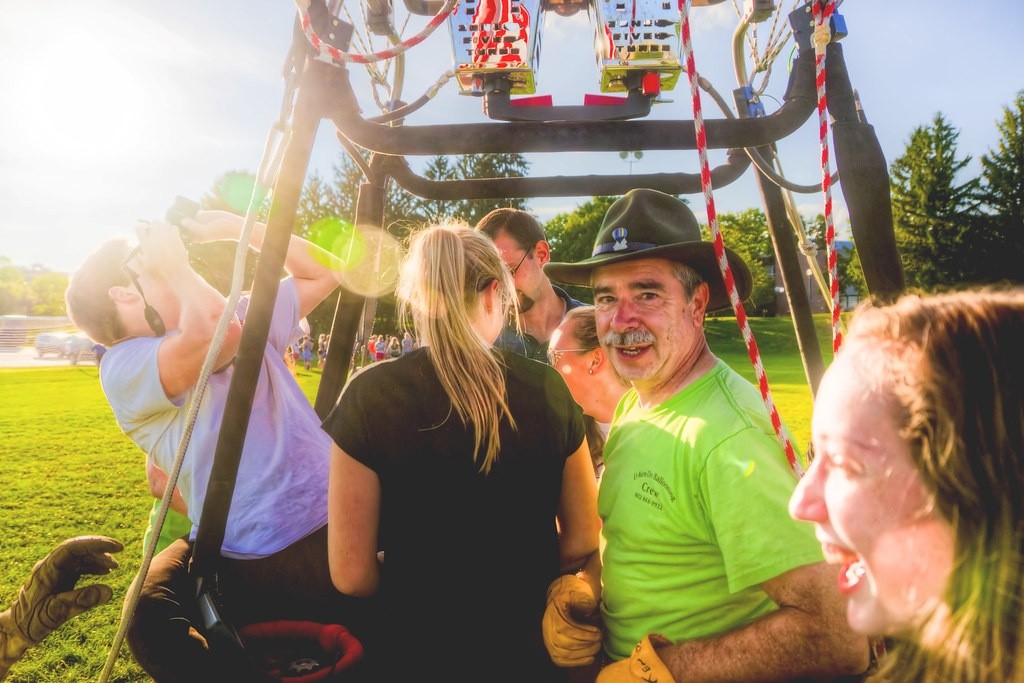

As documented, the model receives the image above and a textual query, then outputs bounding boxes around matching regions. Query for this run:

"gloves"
[541,574,602,667]
[594,632,676,683]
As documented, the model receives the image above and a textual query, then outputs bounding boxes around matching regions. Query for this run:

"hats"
[543,189,752,314]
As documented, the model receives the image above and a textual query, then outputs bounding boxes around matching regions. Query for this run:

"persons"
[279,325,414,376]
[2,533,123,681]
[67,203,347,681]
[787,285,1024,683]
[327,208,634,681]
[543,188,872,680]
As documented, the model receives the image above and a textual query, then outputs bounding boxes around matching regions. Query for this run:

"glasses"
[508,243,533,277]
[547,348,590,367]
